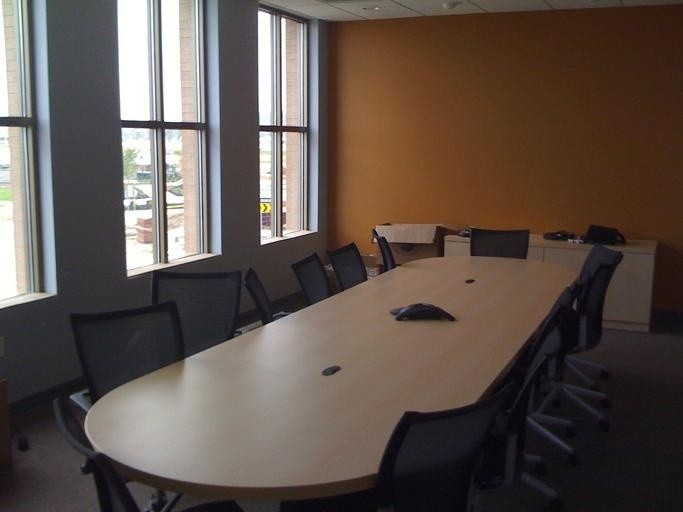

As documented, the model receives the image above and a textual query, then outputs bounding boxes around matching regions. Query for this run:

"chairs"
[332,244,368,289]
[371,400,494,511]
[151,270,243,352]
[244,267,291,322]
[59,301,185,510]
[494,246,623,499]
[293,254,335,305]
[372,236,404,270]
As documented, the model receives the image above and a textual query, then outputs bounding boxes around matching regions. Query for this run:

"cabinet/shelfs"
[438,233,659,333]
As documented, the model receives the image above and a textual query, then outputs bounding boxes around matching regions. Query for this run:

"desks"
[84,256,580,501]
